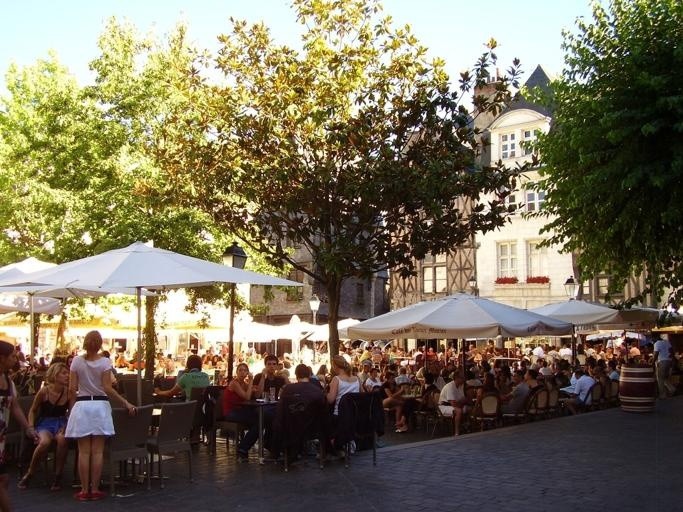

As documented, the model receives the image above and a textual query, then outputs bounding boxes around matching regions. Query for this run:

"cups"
[269,387,275,401]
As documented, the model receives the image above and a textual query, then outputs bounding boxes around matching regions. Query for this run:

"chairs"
[204,385,245,456]
[147,401,194,489]
[9,394,57,481]
[274,394,326,473]
[101,405,151,498]
[149,408,162,481]
[190,386,213,451]
[329,390,379,469]
[0,337,682,434]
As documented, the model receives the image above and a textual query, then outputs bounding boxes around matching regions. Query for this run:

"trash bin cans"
[619,363,658,413]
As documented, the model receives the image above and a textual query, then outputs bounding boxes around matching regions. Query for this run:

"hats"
[362,360,372,366]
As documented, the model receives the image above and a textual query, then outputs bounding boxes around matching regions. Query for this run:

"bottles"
[263,392,269,402]
[415,386,418,394]
[410,391,414,396]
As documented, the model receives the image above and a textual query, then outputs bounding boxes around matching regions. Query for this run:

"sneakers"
[74,491,105,500]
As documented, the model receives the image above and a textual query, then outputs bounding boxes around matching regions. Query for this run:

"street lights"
[307,293,320,366]
[469,276,478,298]
[218,240,249,382]
[562,273,580,365]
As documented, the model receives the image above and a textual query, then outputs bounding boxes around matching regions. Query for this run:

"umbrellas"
[0,257,160,371]
[0,242,305,407]
[0,292,60,314]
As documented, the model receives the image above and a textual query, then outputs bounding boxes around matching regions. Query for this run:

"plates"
[256,399,265,402]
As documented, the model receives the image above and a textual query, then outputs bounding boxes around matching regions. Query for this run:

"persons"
[0,331,682,512]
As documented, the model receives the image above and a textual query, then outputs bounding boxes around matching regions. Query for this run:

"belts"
[77,396,107,400]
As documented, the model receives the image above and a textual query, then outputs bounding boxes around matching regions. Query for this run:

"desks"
[229,398,276,464]
